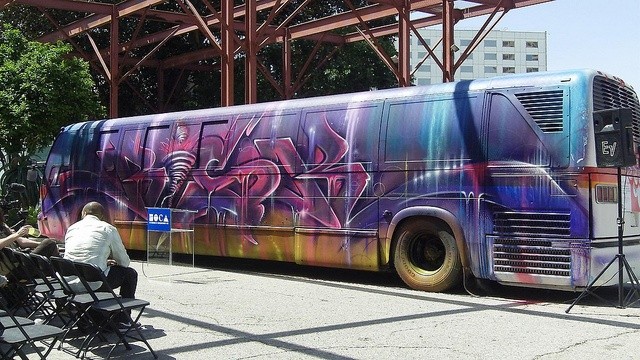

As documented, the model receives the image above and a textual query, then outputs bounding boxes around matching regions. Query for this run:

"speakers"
[593,108,635,168]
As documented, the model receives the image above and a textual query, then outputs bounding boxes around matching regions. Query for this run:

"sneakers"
[118,321,141,333]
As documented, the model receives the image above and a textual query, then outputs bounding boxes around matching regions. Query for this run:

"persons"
[63,202,142,332]
[0,202,63,278]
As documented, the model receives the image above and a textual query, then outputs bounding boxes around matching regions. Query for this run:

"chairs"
[58,262,159,360]
[12,251,67,326]
[0,315,36,359]
[25,252,105,342]
[40,256,132,358]
[1,246,47,316]
[0,275,65,360]
[0,307,8,316]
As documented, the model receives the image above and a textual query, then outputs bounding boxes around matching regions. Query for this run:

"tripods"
[565,175,640,315]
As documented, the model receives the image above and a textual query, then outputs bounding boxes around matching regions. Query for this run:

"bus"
[36,67,640,298]
